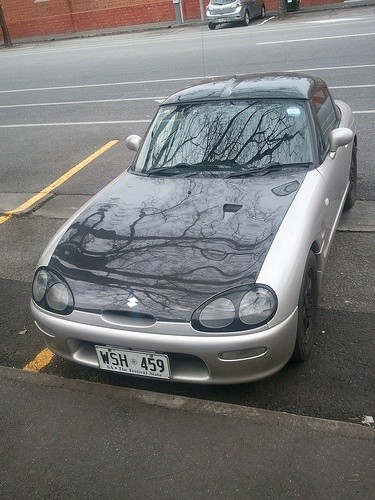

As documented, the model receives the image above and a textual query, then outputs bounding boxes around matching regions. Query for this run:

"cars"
[207,0,265,30]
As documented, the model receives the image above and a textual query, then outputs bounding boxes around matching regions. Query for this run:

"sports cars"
[30,72,357,386]
